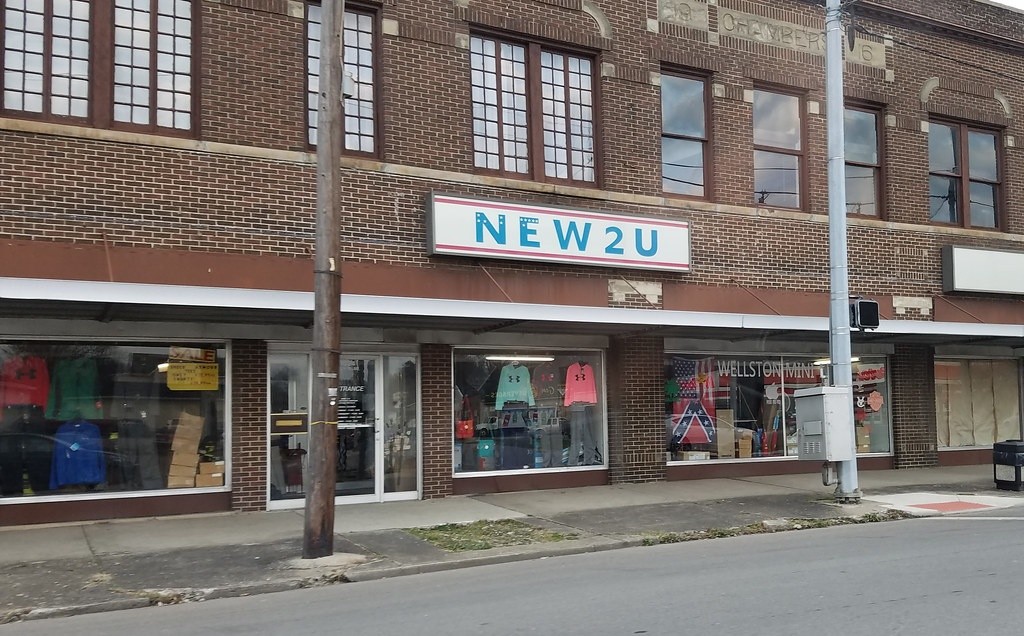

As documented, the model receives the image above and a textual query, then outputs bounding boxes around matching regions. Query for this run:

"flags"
[672,358,716,444]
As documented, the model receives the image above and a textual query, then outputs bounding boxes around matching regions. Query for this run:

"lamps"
[485,354,555,362]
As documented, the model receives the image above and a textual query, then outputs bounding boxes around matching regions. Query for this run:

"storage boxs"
[195,473,224,488]
[199,461,225,474]
[677,450,710,461]
[164,411,205,488]
[855,426,871,452]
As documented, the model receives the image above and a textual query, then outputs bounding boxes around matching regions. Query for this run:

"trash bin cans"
[992,440,1024,491]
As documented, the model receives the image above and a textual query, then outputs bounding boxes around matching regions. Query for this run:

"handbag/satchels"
[478,439,495,457]
[455,419,474,439]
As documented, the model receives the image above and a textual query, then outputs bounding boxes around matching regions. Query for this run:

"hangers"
[578,359,586,366]
[511,360,521,366]
[67,412,82,425]
[542,361,552,366]
